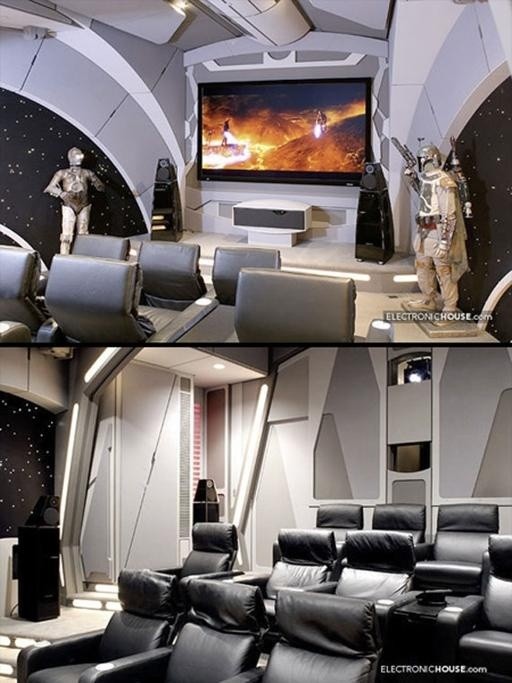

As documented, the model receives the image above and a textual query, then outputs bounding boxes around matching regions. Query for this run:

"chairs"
[0,230,361,345]
[13,497,510,682]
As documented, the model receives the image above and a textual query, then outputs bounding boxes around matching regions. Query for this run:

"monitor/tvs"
[197,76,371,187]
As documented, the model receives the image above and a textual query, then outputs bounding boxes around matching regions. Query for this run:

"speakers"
[355,188,395,265]
[18,527,60,622]
[150,179,184,243]
[193,502,219,526]
[359,162,387,190]
[154,158,177,181]
[194,479,217,502]
[231,199,313,233]
[24,494,59,528]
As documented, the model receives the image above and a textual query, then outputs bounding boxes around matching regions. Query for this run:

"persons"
[221,118,229,145]
[404,142,469,326]
[315,111,328,132]
[44,146,104,256]
